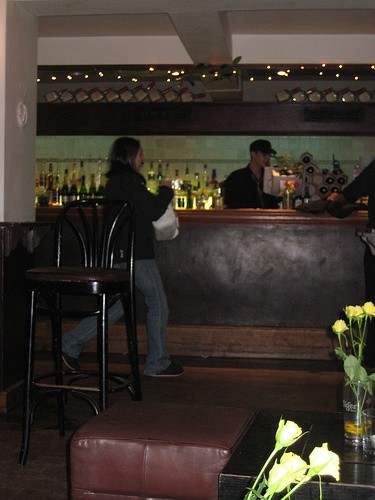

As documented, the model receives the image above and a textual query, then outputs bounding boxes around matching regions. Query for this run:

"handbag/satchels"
[152,203,179,241]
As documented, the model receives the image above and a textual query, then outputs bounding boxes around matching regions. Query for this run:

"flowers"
[331,301,375,428]
[242,416,340,500]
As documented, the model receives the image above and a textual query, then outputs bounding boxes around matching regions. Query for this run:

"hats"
[250,140,277,154]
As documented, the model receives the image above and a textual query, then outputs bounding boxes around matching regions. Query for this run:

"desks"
[218,408,375,500]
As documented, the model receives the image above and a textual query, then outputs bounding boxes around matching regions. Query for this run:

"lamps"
[276,64,287,76]
[171,67,180,76]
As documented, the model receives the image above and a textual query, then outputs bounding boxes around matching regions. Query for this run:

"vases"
[342,372,375,449]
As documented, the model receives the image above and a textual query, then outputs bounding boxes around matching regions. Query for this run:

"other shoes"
[144,361,185,377]
[60,351,81,371]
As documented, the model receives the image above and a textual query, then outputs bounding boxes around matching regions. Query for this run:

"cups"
[361,408,375,455]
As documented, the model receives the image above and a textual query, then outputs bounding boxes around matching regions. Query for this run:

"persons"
[328,158,375,302]
[224,140,284,209]
[62,137,184,377]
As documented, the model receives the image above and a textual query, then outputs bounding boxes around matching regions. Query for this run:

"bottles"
[43,82,206,102]
[303,186,312,204]
[302,155,345,194]
[275,86,375,102]
[35,156,223,209]
[293,194,303,208]
[283,180,293,210]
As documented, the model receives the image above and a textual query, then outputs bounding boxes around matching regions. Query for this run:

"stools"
[70,398,255,500]
[18,197,143,470]
